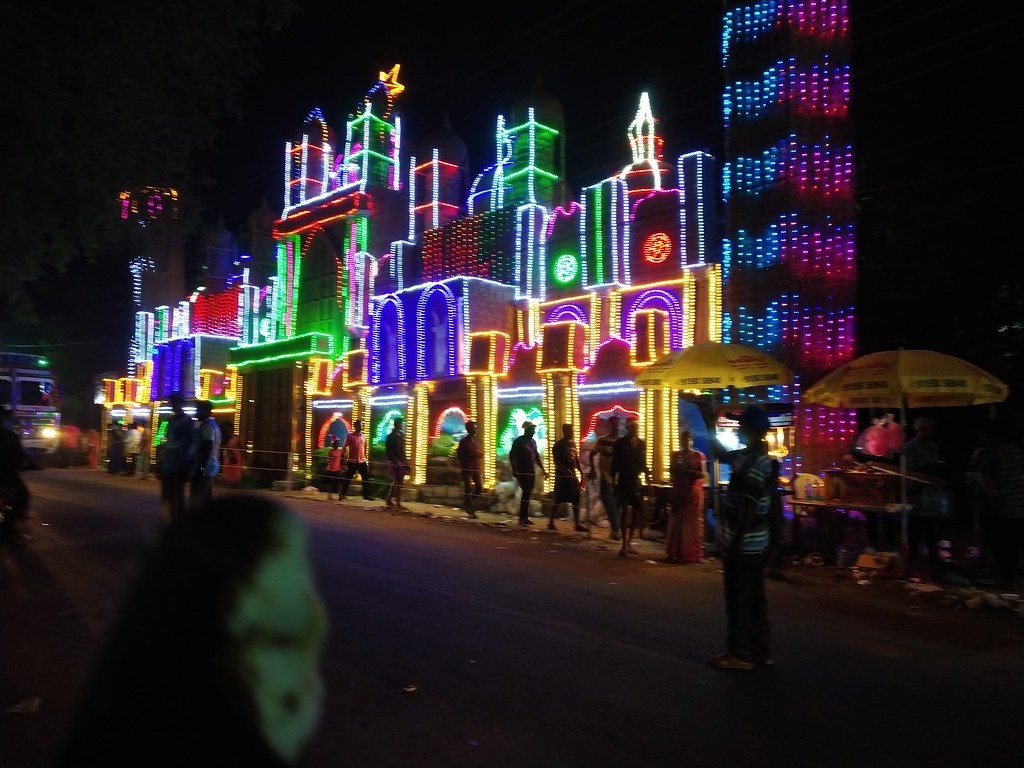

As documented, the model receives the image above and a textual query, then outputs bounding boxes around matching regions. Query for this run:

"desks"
[636,481,914,562]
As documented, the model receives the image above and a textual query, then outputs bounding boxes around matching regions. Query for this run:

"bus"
[0,350,64,471]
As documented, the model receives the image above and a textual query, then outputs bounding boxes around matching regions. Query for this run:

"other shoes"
[338,494,346,500]
[611,532,622,542]
[547,522,557,529]
[363,494,377,500]
[707,651,755,670]
[461,502,478,519]
[520,518,534,526]
[385,496,394,506]
[576,524,590,532]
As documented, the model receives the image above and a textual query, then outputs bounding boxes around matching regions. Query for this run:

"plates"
[1000,593,1021,600]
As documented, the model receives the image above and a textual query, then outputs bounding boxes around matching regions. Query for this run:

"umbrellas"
[638,342,795,550]
[803,347,1009,577]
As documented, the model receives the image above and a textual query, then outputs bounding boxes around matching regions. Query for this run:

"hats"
[725,407,771,431]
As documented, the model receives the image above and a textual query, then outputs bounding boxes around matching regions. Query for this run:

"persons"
[453,421,484,519]
[0,405,35,546]
[906,417,1024,591]
[322,420,376,502]
[510,423,548,526]
[160,392,247,521]
[587,416,654,561]
[56,493,327,768]
[705,406,777,670]
[107,420,150,477]
[665,431,708,563]
[547,424,590,532]
[384,419,409,509]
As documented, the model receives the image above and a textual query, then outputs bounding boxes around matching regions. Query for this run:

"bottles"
[967,535,977,559]
[919,542,929,560]
[840,549,847,566]
[805,479,825,500]
